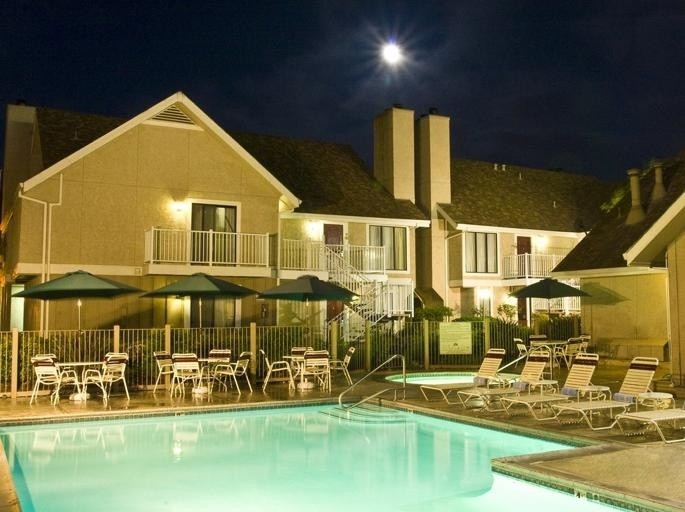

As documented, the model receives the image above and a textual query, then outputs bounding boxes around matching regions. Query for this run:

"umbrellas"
[580,282,631,339]
[9,271,146,331]
[139,272,258,328]
[258,275,360,327]
[508,277,591,321]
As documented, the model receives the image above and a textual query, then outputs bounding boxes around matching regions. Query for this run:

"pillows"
[613,393,636,403]
[560,387,579,396]
[473,376,490,386]
[513,382,530,390]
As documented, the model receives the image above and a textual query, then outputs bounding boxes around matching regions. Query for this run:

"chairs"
[615,400,685,444]
[500,352,600,421]
[259,349,296,392]
[457,350,550,412]
[551,356,659,431]
[324,347,356,388]
[289,347,314,389]
[302,350,331,393]
[514,335,591,370]
[153,349,253,395]
[420,348,506,405]
[29,352,129,406]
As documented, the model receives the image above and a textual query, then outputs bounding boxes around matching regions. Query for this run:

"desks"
[282,355,315,389]
[192,359,229,393]
[533,341,568,368]
[54,362,107,401]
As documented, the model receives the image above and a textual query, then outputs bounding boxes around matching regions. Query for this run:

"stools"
[528,379,559,412]
[577,385,613,421]
[635,392,676,430]
